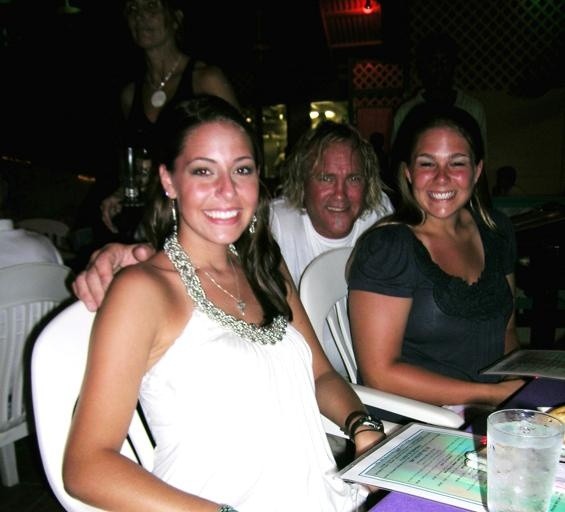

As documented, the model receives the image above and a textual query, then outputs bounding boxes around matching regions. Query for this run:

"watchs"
[340,412,385,439]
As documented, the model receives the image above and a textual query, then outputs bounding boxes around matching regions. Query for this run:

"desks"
[370,377,565,512]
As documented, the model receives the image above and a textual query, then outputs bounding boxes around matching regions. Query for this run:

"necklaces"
[147,53,183,108]
[193,253,246,314]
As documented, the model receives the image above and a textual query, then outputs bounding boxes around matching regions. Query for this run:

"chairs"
[301,246,465,428]
[1,262,76,489]
[32,284,406,512]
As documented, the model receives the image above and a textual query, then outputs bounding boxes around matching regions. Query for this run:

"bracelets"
[352,428,383,437]
[217,504,238,512]
[344,412,367,428]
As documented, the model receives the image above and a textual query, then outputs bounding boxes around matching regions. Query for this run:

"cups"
[486,408,565,512]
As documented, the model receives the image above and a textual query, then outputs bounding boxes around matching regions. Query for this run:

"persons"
[348,104,528,407]
[63,93,386,512]
[99,0,240,244]
[70,120,394,471]
[385,33,494,209]
